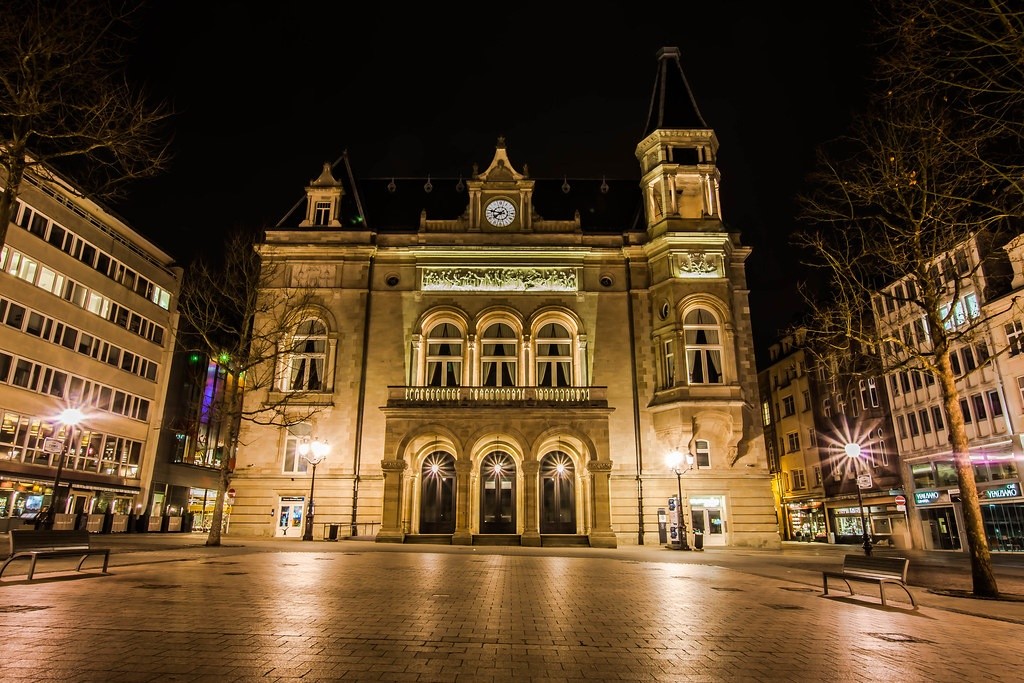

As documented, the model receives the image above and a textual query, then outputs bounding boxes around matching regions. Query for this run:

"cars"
[0,506,23,517]
[19,511,48,530]
[997,523,1024,551]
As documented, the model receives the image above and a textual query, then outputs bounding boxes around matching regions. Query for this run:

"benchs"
[0,529,111,580]
[822,554,920,607]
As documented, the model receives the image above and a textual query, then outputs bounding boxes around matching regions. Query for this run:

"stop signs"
[895,496,905,505]
[227,489,235,498]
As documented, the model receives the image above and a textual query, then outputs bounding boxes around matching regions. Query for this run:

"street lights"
[41,409,77,530]
[299,442,329,541]
[846,444,873,556]
[666,451,694,550]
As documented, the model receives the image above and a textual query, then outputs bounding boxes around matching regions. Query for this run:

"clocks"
[485,199,517,228]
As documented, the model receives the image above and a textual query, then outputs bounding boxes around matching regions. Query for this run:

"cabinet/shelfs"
[839,518,842,532]
[791,517,801,532]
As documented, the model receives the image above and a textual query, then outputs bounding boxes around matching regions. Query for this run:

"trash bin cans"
[35,505,55,531]
[826,532,836,544]
[692,528,705,552]
[327,524,339,542]
